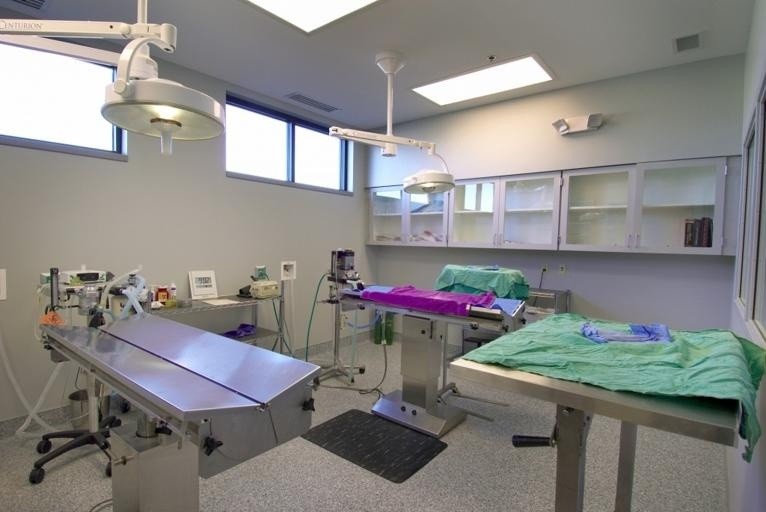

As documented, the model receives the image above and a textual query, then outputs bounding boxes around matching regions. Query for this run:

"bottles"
[170,283,177,300]
[156,288,168,305]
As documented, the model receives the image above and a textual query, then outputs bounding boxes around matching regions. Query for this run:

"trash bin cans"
[374,309,393,345]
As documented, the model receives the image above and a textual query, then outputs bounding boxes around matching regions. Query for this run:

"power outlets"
[255,265,267,279]
[542,262,549,272]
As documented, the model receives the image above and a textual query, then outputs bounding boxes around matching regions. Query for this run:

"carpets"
[301,409,448,484]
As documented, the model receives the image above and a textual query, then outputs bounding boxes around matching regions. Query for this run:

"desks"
[447,312,744,511]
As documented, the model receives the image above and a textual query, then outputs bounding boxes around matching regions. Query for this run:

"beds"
[336,280,525,437]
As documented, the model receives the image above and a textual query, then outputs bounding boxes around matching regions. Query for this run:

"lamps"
[552,113,602,136]
[99,0,225,158]
[329,52,454,194]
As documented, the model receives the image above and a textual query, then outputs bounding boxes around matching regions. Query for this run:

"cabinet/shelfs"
[366,158,740,258]
[523,287,572,321]
